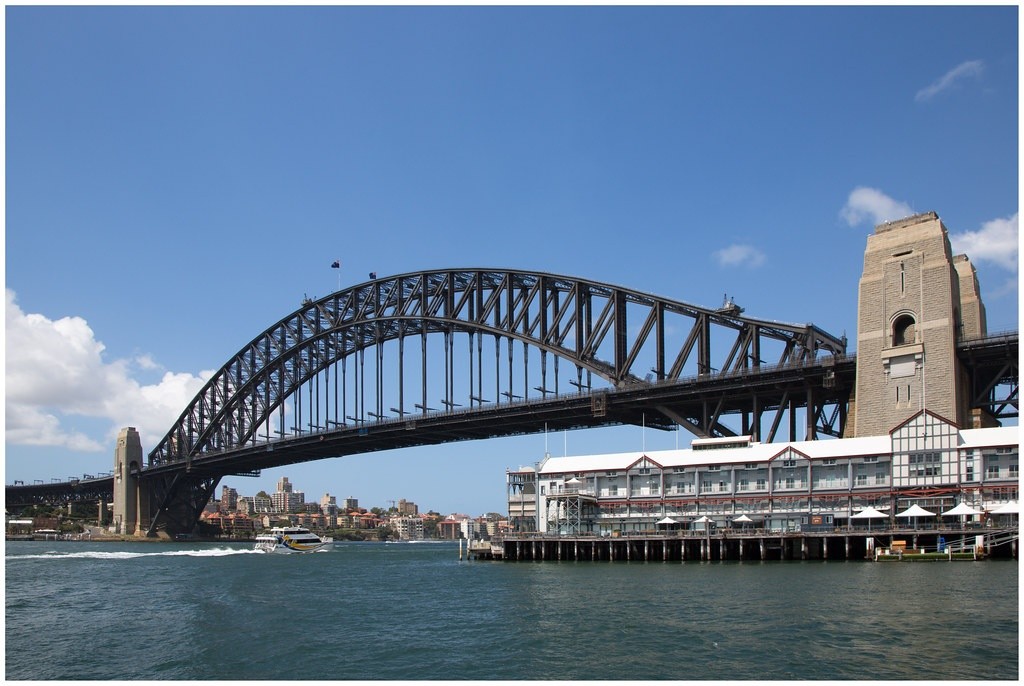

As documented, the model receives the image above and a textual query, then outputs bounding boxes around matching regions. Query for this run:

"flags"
[332,259,340,268]
[369,272,376,279]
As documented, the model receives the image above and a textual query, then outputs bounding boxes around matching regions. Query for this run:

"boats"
[863,542,979,561]
[254,525,336,556]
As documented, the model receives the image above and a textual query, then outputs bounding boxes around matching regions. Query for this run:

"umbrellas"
[941,502,985,525]
[732,515,752,528]
[992,502,1018,529]
[894,504,937,529]
[694,516,714,529]
[565,478,582,489]
[849,507,889,531]
[655,517,680,534]
